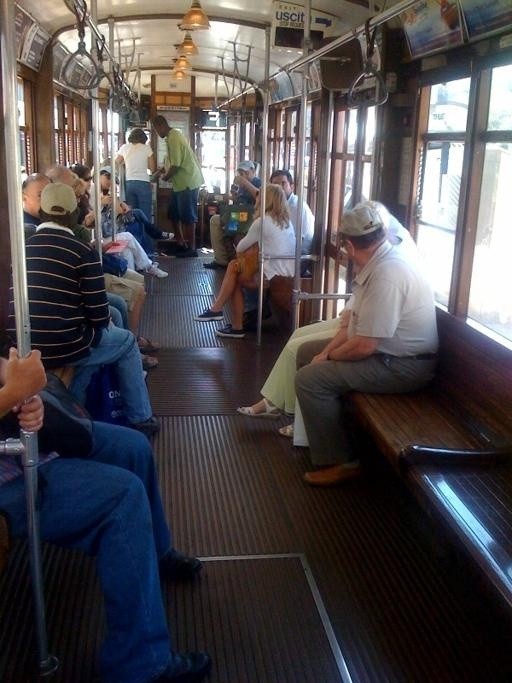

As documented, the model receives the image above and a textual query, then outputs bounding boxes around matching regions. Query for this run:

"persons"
[270,170,316,277]
[236,202,434,438]
[23,174,146,382]
[295,204,438,488]
[6,182,160,441]
[67,163,169,279]
[44,164,162,369]
[151,114,205,258]
[0,349,214,683]
[90,167,160,269]
[204,161,266,269]
[192,183,296,338]
[94,158,175,257]
[115,129,157,222]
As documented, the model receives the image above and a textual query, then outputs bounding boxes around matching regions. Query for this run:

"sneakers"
[154,231,174,243]
[139,265,168,280]
[194,307,225,322]
[135,337,159,352]
[130,417,161,440]
[175,248,198,258]
[140,355,159,370]
[166,246,188,256]
[216,324,246,338]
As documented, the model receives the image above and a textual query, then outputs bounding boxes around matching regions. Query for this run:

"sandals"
[279,424,294,438]
[236,398,281,422]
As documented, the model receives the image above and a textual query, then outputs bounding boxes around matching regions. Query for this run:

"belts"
[396,352,439,361]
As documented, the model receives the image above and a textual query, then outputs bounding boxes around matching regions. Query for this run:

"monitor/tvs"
[199,109,228,130]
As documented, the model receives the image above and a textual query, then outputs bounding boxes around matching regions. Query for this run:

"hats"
[236,160,256,172]
[335,206,383,236]
[100,165,120,185]
[40,181,77,215]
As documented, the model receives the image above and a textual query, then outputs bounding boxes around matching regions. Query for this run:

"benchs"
[400,444,512,609]
[350,300,512,490]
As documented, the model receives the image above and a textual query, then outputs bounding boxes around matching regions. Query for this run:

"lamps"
[177,31,198,56]
[180,1,212,31]
[174,71,186,80]
[174,56,192,70]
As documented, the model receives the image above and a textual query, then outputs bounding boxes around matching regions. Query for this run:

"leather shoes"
[203,261,227,269]
[162,556,202,583]
[302,465,368,488]
[152,648,213,682]
